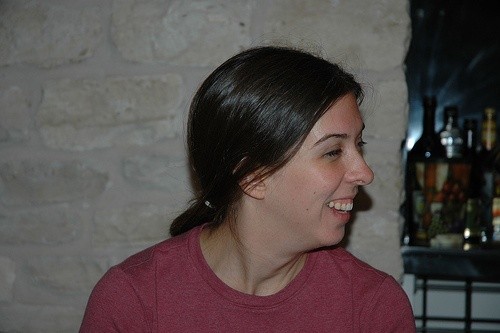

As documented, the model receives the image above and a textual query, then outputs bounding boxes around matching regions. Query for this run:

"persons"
[79,42,416,333]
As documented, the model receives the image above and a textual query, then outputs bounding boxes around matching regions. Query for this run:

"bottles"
[448,118,487,242]
[437,105,461,158]
[405,95,447,247]
[477,108,500,249]
